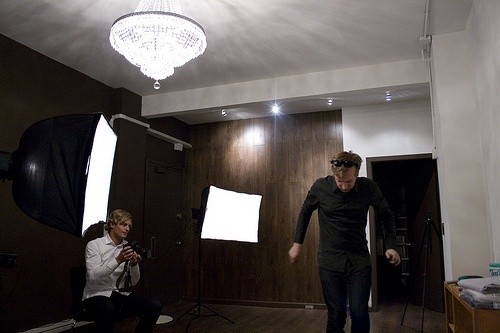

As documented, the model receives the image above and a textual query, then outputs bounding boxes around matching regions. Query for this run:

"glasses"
[330,160,360,170]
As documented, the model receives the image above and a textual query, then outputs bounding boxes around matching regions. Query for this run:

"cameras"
[122,241,149,259]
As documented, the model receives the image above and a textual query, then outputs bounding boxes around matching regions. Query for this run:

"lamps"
[221,109,228,118]
[385,90,392,101]
[107,0,209,90]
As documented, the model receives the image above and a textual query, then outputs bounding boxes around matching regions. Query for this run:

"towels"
[457,276,500,309]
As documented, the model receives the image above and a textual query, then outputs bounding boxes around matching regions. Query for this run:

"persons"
[82,209,150,333]
[289,150,401,333]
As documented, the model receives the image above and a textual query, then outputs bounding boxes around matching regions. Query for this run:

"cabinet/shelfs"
[443,280,500,333]
